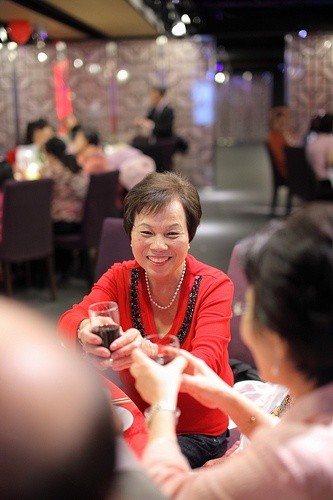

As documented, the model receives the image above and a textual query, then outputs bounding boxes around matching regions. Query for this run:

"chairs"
[92,218,134,284]
[0,179,57,303]
[55,169,120,288]
[262,127,333,216]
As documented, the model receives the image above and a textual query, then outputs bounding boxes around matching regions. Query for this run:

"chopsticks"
[112,398,131,405]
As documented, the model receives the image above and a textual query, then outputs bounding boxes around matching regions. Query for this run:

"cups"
[88,301,120,366]
[141,333,180,366]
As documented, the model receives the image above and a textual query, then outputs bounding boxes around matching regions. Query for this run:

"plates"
[114,406,134,431]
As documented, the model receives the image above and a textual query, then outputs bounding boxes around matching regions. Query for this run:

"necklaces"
[145,259,187,311]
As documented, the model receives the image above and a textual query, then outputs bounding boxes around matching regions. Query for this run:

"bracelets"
[77,317,90,347]
[142,403,180,430]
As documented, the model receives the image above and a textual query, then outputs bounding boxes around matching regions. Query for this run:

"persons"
[0,294,167,500]
[57,173,233,468]
[130,202,333,500]
[9,82,176,233]
[264,111,333,203]
